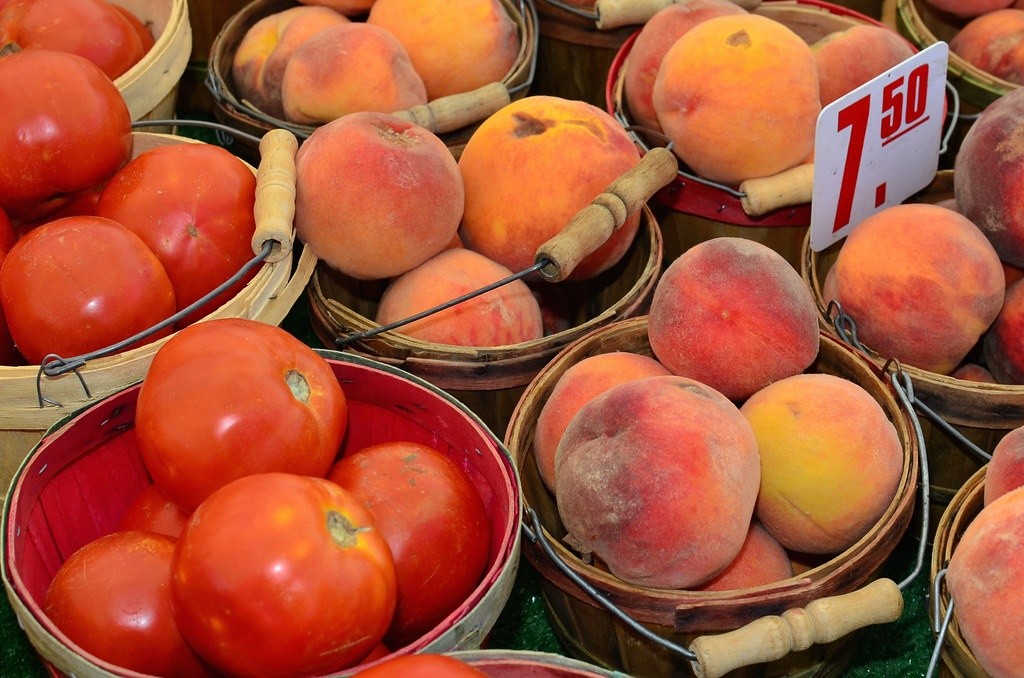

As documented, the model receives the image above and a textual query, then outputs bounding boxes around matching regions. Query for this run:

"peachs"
[239,0,1024,678]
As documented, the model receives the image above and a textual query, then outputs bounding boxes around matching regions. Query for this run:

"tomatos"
[0,0,487,678]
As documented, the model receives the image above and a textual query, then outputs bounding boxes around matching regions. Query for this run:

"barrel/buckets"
[0,348,525,678]
[299,145,663,445]
[344,649,629,678]
[535,0,880,113]
[881,0,1024,126]
[0,0,195,135]
[600,0,960,275]
[795,171,1024,517]
[503,316,930,678]
[203,0,540,167]
[925,460,1024,678]
[0,118,299,509]
